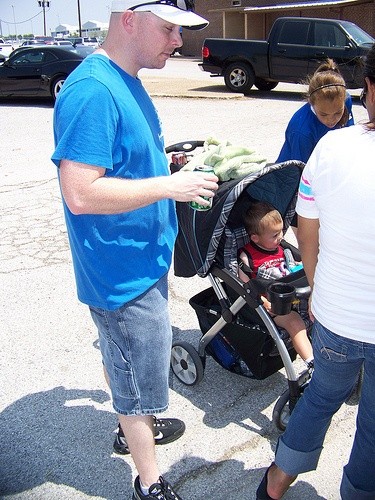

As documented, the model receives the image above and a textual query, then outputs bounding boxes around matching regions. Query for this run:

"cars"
[0,32,101,62]
[0,47,99,104]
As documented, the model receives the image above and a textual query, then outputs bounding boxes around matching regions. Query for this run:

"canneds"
[171,151,188,165]
[189,166,215,212]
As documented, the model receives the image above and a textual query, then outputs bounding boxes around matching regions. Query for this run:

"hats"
[111,0,210,32]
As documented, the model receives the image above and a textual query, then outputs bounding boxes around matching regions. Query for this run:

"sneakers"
[132,475,182,500]
[114,418,186,454]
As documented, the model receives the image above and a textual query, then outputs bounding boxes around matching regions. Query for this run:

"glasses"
[128,0,196,11]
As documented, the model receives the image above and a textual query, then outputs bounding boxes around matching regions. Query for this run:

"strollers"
[162,140,366,439]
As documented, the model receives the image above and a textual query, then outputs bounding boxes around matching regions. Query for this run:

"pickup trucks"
[198,16,375,92]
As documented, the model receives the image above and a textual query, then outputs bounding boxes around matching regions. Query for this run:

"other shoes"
[256,462,278,500]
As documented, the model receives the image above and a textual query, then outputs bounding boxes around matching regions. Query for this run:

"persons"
[238,44,375,500]
[50,0,219,500]
